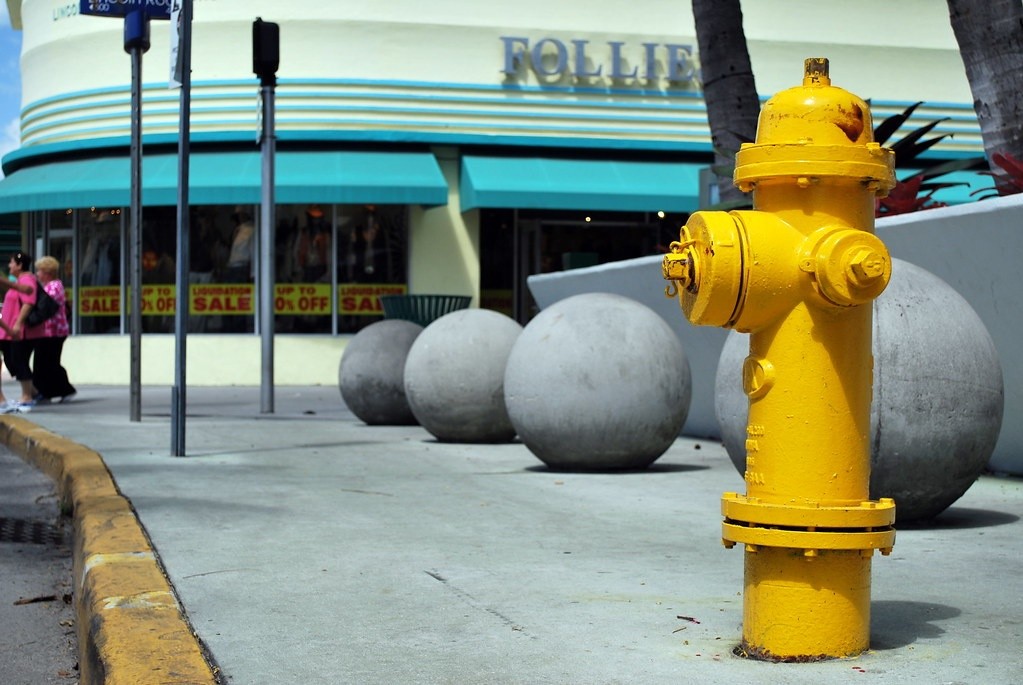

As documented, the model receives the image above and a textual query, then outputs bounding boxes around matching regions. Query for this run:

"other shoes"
[33,394,51,407]
[0,404,12,414]
[15,398,37,412]
[59,388,75,404]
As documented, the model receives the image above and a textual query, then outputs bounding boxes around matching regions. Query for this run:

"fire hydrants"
[661,57,896,662]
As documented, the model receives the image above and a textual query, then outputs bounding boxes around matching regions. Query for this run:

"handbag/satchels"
[18,281,59,330]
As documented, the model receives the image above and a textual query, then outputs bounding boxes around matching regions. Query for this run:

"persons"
[31,256,76,405]
[0,270,34,296]
[82,219,121,333]
[0,251,44,406]
[0,318,17,408]
[189,203,256,333]
[275,216,392,284]
